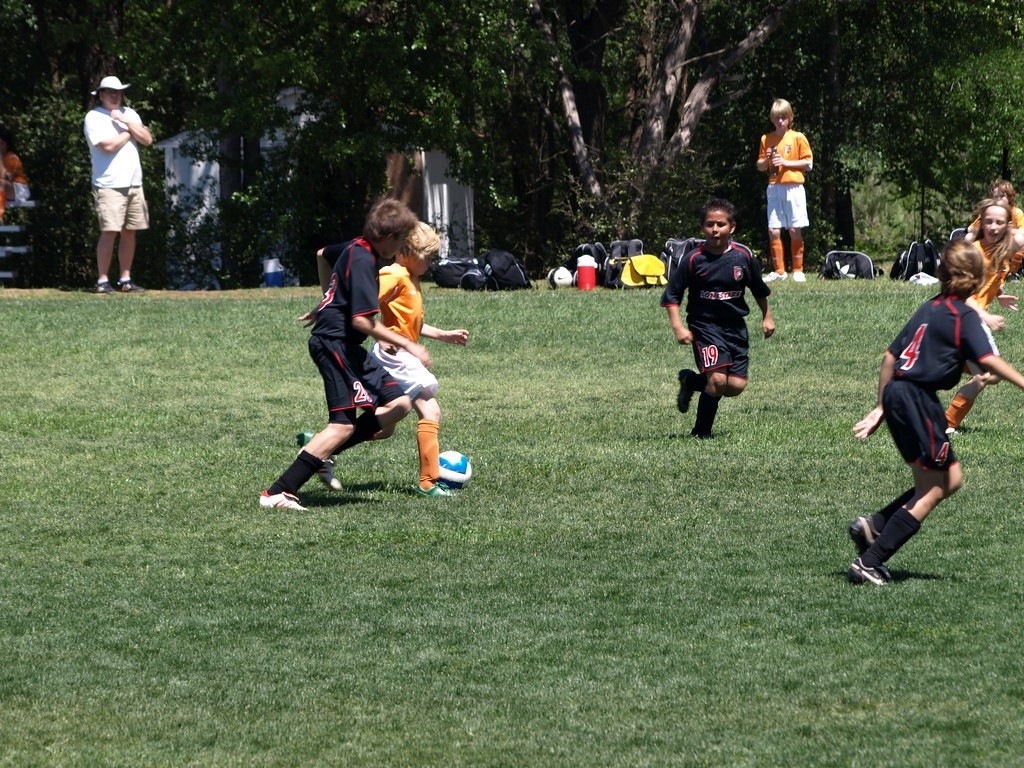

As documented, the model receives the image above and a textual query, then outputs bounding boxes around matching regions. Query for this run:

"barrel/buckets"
[263,270,282,287]
[577,255,598,290]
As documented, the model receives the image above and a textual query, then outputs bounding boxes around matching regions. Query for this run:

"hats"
[91,76,131,99]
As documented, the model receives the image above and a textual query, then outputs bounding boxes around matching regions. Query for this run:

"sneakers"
[792,271,806,284]
[415,481,457,498]
[296,431,315,448]
[116,280,144,293]
[848,556,894,586]
[95,282,114,293]
[259,490,309,511]
[762,270,788,284]
[319,459,344,491]
[850,516,880,556]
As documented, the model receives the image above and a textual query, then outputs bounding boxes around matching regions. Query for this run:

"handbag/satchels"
[606,238,696,289]
[818,250,884,281]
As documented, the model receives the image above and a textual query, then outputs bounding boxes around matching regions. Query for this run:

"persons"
[756,98,813,282]
[967,180,1023,281]
[0,124,26,219]
[260,197,430,512]
[851,241,1024,584]
[317,222,469,496]
[658,199,775,437]
[85,76,153,293]
[946,202,1019,433]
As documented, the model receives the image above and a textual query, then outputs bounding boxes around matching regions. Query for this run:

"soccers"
[436,450,473,489]
[547,266,574,289]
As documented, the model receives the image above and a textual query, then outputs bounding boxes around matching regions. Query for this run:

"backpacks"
[572,242,608,287]
[890,239,938,282]
[430,250,534,290]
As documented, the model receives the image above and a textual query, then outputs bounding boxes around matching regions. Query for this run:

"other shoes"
[690,428,714,439]
[677,369,695,413]
